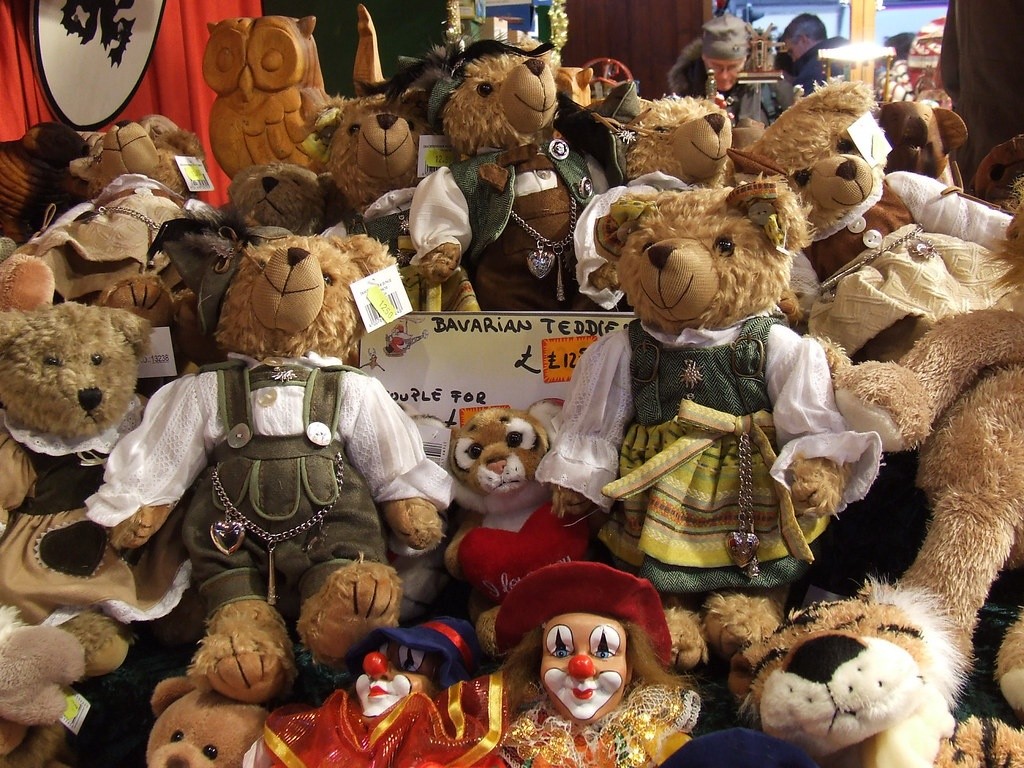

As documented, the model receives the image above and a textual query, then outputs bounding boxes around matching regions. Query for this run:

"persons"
[665,14,794,129]
[776,13,870,96]
[481,560,696,768]
[253,615,510,768]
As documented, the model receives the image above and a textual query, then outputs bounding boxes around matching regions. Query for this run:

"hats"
[702,14,748,59]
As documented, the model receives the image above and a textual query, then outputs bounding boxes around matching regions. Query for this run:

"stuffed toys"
[0,37,1024,768]
